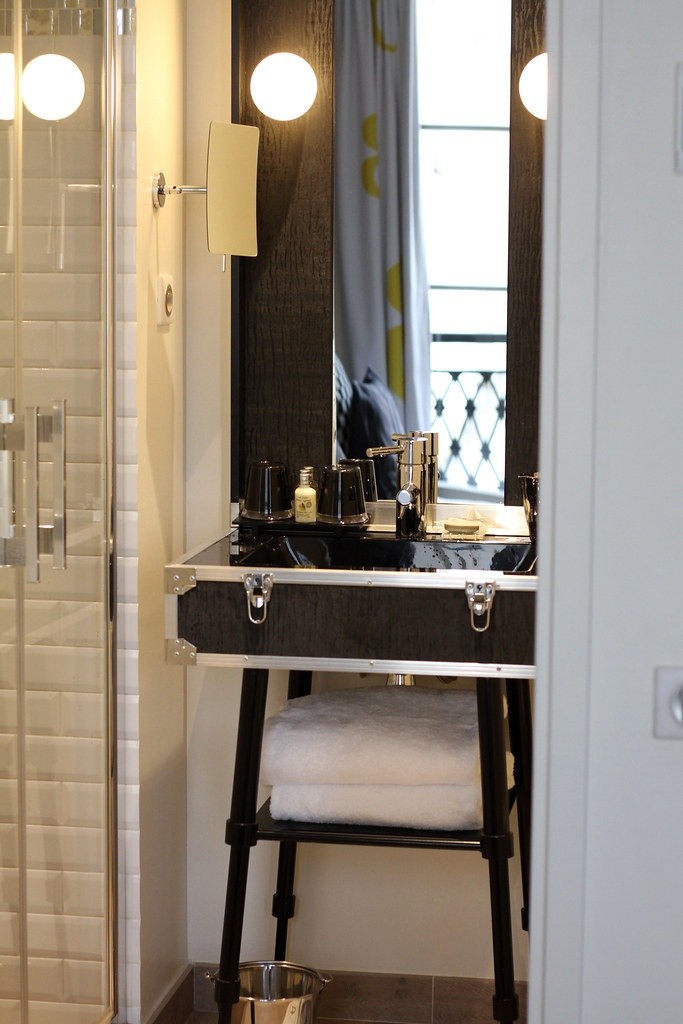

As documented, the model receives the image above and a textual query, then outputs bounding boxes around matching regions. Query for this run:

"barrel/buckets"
[203,959,333,1024]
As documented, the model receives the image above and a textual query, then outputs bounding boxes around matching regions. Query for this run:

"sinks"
[233,524,541,591]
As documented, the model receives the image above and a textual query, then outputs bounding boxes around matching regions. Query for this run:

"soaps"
[443,518,482,535]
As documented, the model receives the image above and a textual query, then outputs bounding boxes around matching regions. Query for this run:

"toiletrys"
[303,464,320,495]
[293,469,319,524]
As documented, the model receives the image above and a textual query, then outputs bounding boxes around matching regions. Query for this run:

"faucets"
[366,437,432,537]
[390,429,445,510]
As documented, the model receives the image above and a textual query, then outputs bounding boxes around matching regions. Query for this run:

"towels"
[262,683,508,792]
[271,750,515,838]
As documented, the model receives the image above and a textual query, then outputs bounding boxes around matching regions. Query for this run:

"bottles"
[294,470,317,523]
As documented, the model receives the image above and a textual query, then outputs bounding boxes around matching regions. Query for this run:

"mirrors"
[229,1,546,508]
